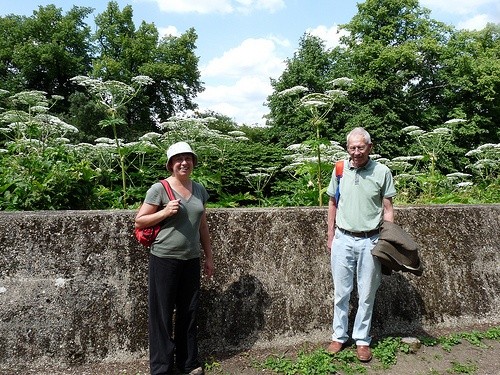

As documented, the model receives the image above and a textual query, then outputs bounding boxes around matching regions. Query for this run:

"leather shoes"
[356,345,372,362]
[327,340,342,355]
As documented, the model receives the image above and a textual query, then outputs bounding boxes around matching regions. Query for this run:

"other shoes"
[188,365,205,375]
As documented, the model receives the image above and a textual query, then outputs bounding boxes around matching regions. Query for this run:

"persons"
[134,142,215,375]
[326,125,397,362]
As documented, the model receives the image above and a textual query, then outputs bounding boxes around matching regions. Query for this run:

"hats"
[166,142,198,172]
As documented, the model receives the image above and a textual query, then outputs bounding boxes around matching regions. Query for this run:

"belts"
[338,226,383,239]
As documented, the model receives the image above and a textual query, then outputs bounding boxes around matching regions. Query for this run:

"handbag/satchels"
[136,179,177,248]
